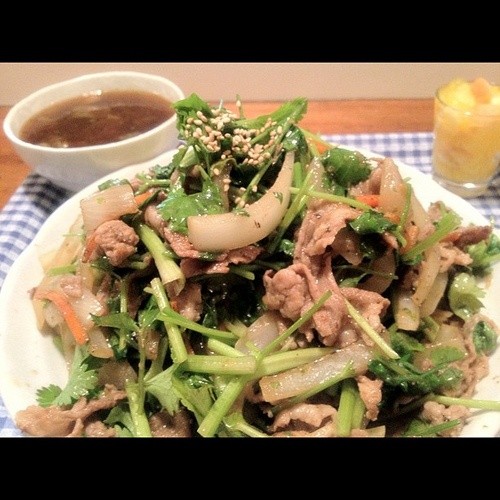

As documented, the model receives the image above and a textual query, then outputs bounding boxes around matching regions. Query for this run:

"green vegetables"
[30,90,500,438]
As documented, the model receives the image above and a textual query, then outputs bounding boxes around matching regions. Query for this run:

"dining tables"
[1,96,500,438]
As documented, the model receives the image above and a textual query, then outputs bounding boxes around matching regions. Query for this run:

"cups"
[433,86,500,197]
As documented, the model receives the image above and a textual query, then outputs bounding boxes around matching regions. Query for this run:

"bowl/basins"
[3,72,186,192]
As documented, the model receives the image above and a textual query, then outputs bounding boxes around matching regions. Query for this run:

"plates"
[0,143,500,437]
